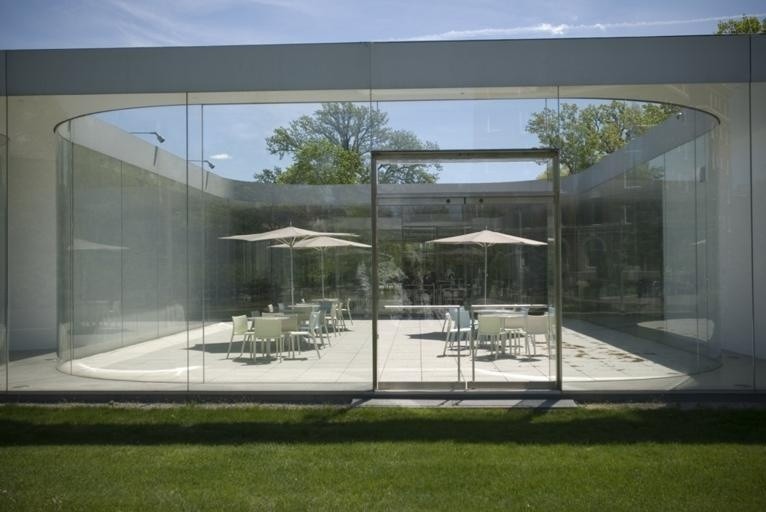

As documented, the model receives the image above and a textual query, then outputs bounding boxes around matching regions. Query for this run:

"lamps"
[187,159,215,170]
[129,131,165,144]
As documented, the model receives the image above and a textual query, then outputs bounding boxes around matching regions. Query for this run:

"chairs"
[441,303,557,362]
[225,297,354,363]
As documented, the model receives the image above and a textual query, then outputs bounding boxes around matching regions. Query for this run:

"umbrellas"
[216,222,359,310]
[265,235,373,299]
[424,227,551,303]
